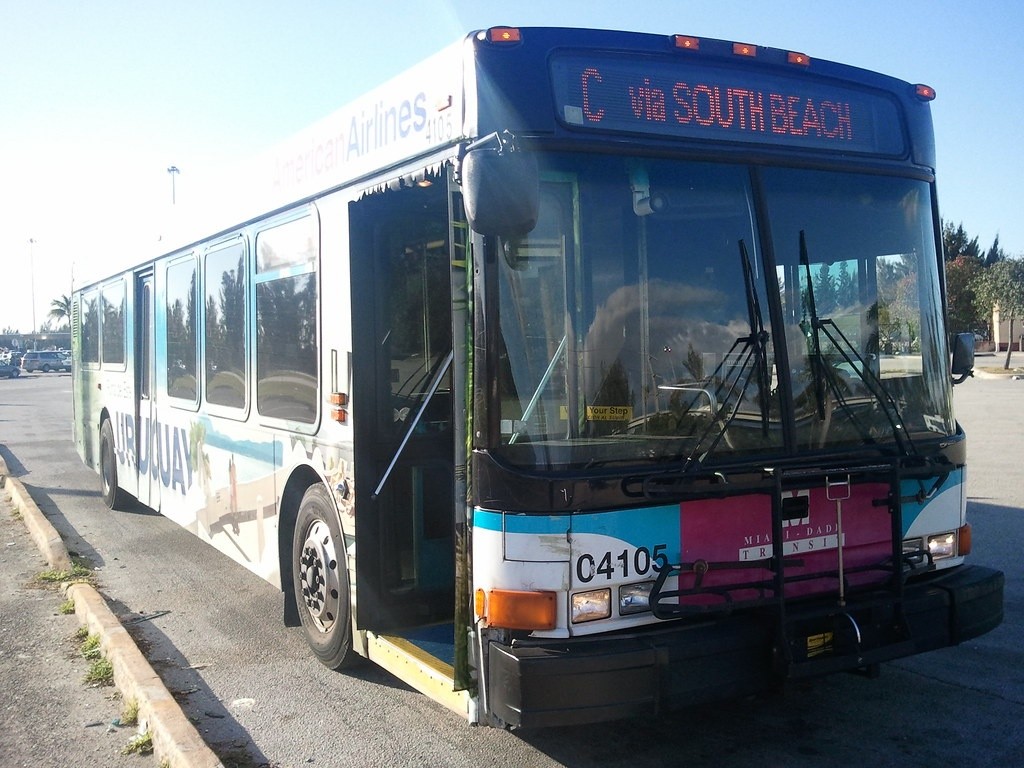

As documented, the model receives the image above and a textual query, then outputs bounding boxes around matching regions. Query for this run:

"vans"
[22,351,68,372]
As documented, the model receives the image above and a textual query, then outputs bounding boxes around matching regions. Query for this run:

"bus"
[65,22,1006,744]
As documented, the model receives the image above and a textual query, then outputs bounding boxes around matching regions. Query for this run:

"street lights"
[27,239,39,333]
[166,165,180,205]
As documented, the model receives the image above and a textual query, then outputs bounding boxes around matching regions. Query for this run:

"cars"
[0,360,21,378]
[0,347,22,366]
